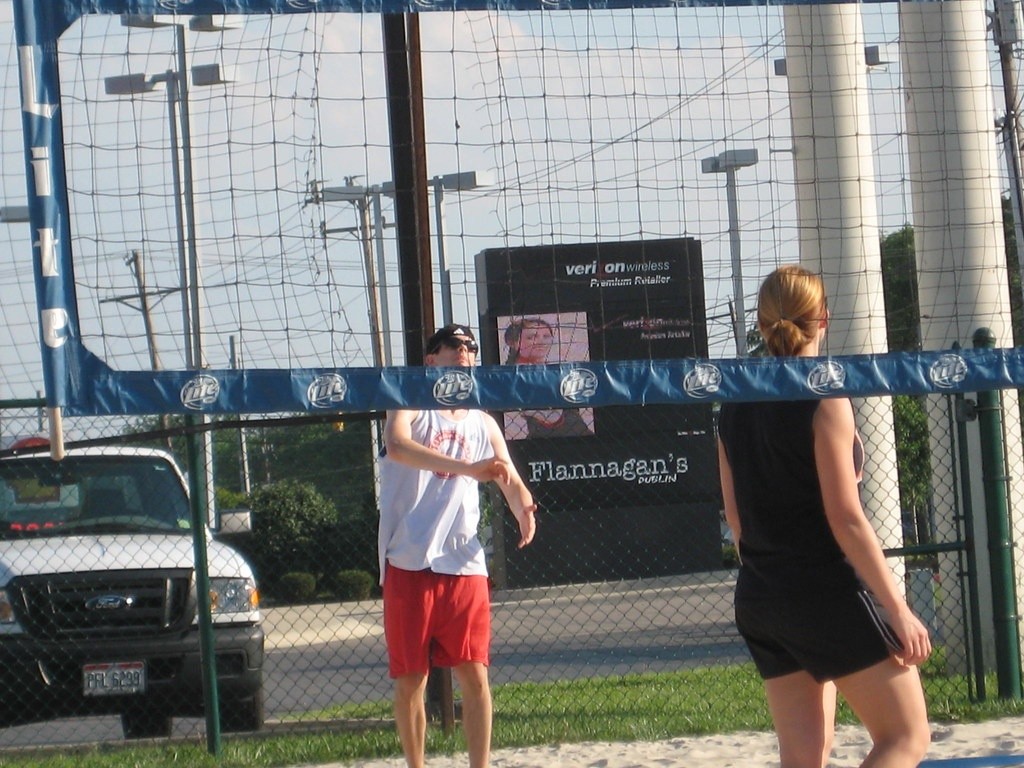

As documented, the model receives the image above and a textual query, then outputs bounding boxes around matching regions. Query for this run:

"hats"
[436,324,473,335]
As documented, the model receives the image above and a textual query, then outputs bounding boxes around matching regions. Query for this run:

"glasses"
[431,336,478,358]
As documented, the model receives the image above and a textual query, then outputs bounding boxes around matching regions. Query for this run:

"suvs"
[0,446,269,735]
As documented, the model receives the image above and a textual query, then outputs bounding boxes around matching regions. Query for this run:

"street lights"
[381,169,495,328]
[120,14,245,530]
[320,182,399,370]
[700,149,760,355]
[102,60,237,369]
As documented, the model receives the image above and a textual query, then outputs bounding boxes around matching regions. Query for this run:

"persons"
[379,323,537,768]
[504,318,593,437]
[718,266,931,768]
